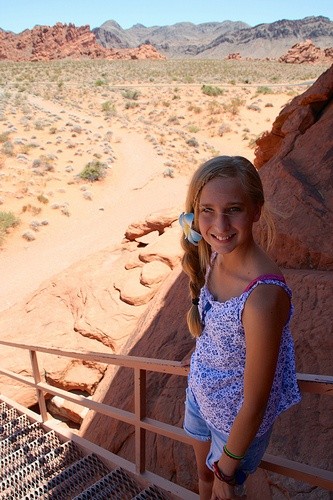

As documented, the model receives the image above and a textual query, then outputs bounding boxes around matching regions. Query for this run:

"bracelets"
[223,444,245,462]
[211,459,246,486]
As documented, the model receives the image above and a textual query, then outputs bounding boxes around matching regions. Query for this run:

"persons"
[181,156,292,500]
